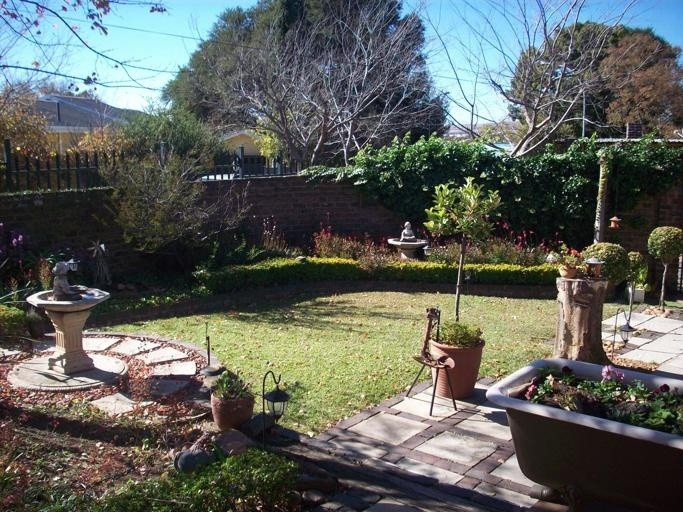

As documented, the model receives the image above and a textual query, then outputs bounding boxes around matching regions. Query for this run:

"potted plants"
[627,254,648,302]
[430,317,486,398]
[210,372,253,432]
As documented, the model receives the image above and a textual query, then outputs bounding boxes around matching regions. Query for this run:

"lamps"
[262,372,289,451]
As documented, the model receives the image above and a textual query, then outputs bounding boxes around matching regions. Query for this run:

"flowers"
[525,359,671,422]
[547,240,580,269]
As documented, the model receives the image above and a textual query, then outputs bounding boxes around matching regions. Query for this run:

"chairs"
[405,306,456,415]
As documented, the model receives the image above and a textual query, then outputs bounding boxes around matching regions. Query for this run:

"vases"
[559,266,576,278]
